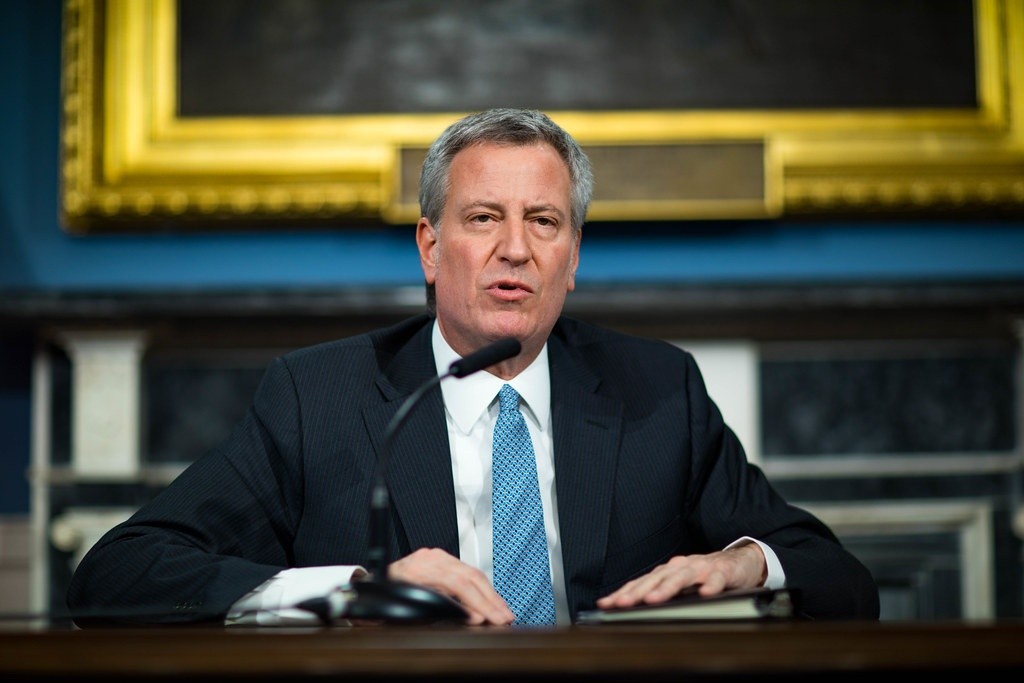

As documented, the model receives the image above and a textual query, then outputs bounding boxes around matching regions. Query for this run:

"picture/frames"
[59,0,1024,230]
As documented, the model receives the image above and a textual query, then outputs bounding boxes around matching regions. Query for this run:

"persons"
[71,109,879,633]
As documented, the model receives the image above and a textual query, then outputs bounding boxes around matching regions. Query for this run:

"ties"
[489,385,557,627]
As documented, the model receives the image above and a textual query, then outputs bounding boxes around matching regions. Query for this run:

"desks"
[0,615,1024,683]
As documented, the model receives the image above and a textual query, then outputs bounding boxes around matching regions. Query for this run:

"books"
[575,589,791,624]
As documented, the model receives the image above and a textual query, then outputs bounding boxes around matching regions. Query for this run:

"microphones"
[342,336,523,623]
